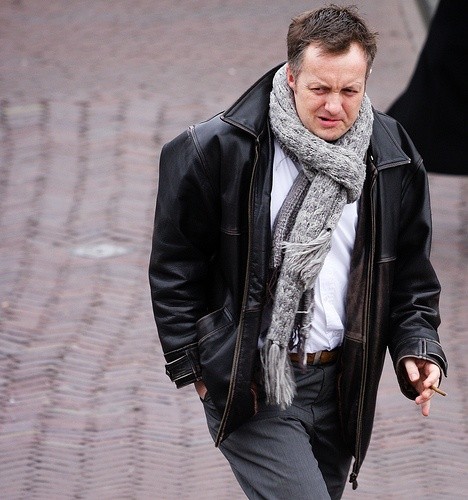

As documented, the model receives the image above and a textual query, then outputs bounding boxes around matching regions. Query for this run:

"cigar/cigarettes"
[428,385,447,397]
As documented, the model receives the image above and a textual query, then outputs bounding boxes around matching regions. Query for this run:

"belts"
[291,349,339,366]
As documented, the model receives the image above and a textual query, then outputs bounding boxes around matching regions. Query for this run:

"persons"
[147,2,445,500]
